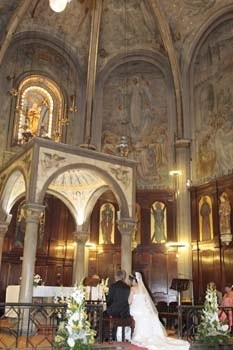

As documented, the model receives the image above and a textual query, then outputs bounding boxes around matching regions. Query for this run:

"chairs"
[156,301,192,330]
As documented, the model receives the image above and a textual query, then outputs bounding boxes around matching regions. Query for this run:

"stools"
[100,315,135,345]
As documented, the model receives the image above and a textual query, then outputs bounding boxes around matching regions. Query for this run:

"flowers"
[193,281,230,346]
[55,287,97,350]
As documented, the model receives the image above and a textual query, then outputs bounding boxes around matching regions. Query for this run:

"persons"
[99,271,133,342]
[152,202,165,243]
[102,204,113,244]
[200,198,212,242]
[127,272,190,350]
[219,193,231,234]
[217,282,233,333]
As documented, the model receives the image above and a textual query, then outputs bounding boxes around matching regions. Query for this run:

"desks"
[5,285,107,318]
